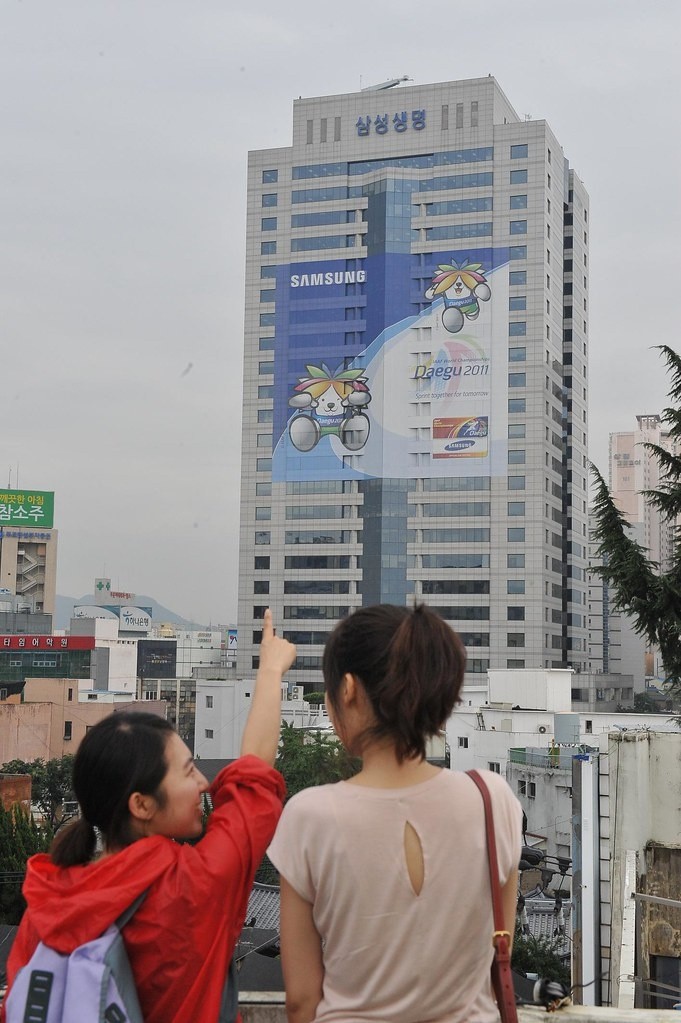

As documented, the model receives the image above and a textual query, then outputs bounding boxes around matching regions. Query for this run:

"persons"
[0,609,297,1022]
[264,602,525,1023]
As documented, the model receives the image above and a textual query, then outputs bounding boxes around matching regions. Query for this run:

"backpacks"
[5,885,239,1023]
